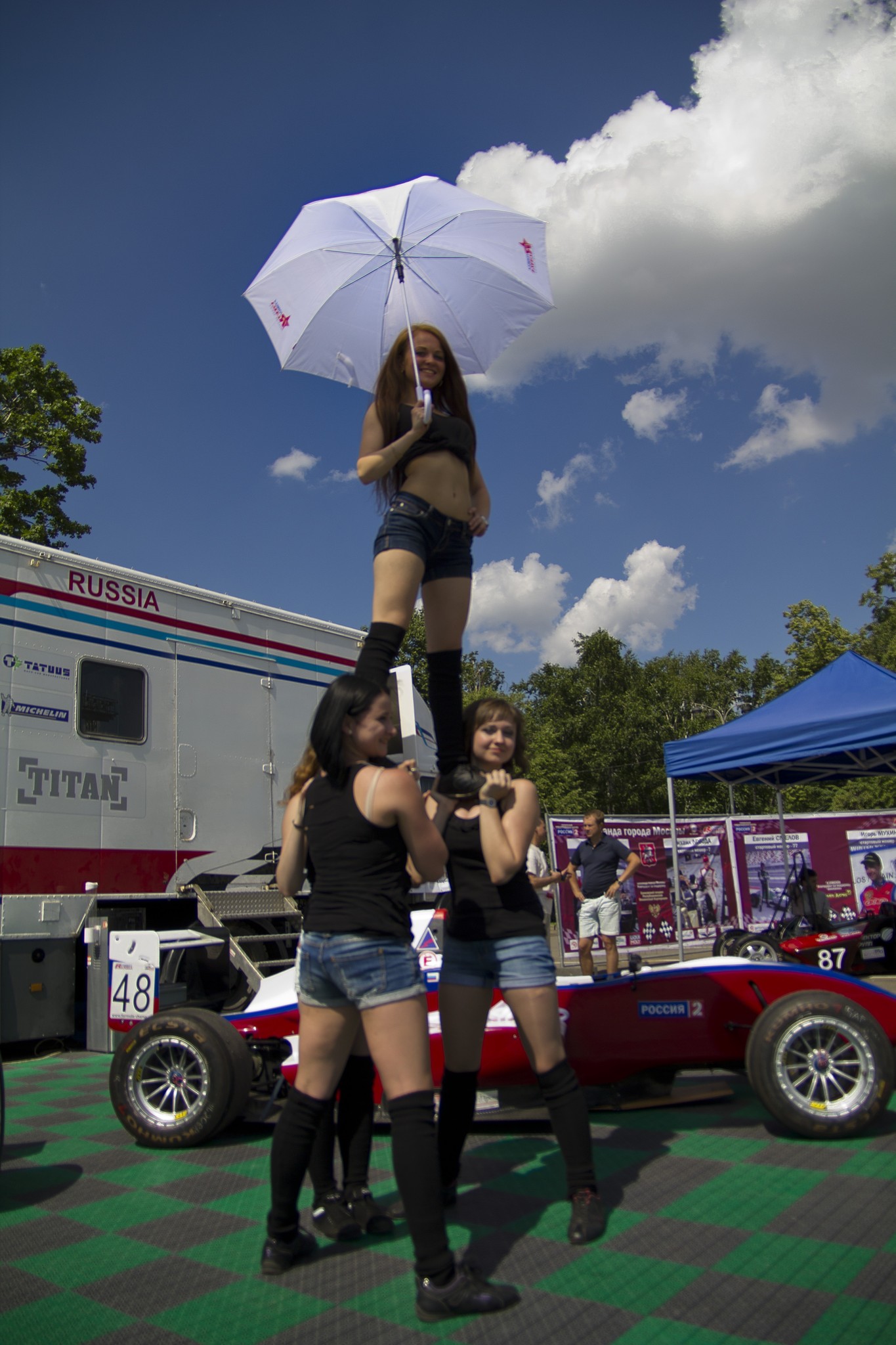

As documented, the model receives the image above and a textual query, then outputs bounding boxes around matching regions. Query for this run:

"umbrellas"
[237,173,556,425]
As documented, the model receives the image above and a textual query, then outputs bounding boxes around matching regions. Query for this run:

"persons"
[261,675,524,1323]
[680,854,719,929]
[790,869,830,920]
[526,818,573,956]
[566,808,642,977]
[758,863,773,912]
[401,697,605,1248]
[356,325,495,799]
[286,747,396,1248]
[858,851,896,918]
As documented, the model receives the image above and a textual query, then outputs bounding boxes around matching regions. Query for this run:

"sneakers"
[436,764,486,798]
[566,1188,606,1244]
[416,1266,520,1322]
[261,1233,317,1274]
[310,1191,362,1241]
[384,1184,457,1216]
[345,1189,395,1233]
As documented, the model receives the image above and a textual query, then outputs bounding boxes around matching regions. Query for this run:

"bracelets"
[480,514,488,527]
[617,878,623,885]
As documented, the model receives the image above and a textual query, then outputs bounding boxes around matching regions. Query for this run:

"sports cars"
[707,903,896,977]
[98,910,896,1139]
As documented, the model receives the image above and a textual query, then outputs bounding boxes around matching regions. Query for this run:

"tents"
[662,650,896,961]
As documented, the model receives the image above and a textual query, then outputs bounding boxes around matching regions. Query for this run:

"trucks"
[0,535,437,1050]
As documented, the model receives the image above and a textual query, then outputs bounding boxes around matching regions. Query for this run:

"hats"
[861,852,881,865]
[703,856,710,863]
